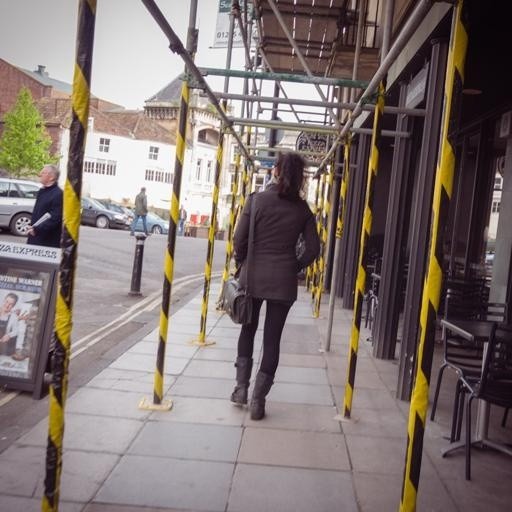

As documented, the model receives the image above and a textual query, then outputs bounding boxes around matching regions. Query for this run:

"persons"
[229,148,321,421]
[1,290,33,359]
[129,184,150,238]
[25,163,64,249]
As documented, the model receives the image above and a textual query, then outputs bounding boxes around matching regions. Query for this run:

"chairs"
[364,255,511,481]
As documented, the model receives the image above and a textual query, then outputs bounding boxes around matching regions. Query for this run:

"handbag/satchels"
[220,278,255,327]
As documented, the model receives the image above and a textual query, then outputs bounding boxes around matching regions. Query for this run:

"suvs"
[1,177,46,237]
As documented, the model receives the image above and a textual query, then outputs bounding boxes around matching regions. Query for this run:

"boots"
[249,368,276,422]
[230,355,254,405]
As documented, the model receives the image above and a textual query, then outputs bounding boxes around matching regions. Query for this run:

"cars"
[80,197,171,235]
